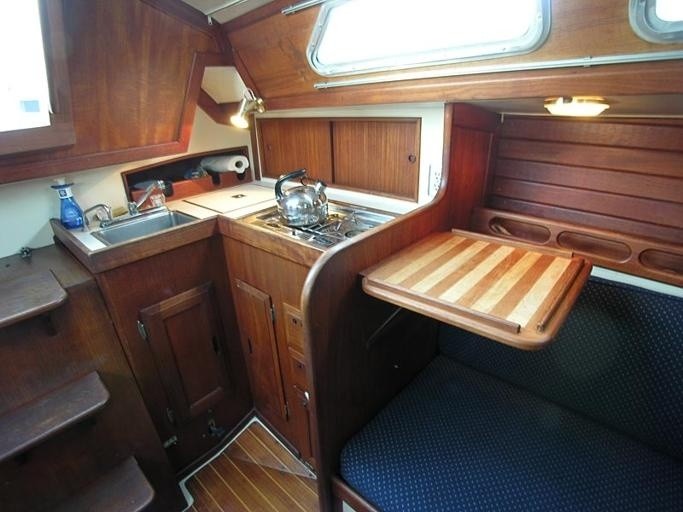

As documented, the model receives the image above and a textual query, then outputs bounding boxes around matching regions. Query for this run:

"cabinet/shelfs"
[220,234,314,467]
[55,234,252,470]
[0,247,188,512]
[255,115,424,203]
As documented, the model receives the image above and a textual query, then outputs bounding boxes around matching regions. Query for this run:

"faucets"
[127,179,165,216]
[82,204,112,230]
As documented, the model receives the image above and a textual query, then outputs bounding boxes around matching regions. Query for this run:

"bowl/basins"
[133,179,173,195]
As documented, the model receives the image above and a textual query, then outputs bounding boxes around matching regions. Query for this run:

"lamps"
[229,87,264,130]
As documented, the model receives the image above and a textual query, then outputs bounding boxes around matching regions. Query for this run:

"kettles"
[273,169,330,228]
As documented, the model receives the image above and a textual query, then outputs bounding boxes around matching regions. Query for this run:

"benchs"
[300,99,683,512]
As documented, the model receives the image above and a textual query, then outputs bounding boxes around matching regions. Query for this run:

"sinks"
[90,208,204,246]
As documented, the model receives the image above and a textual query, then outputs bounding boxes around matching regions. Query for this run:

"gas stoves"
[254,194,395,259]
